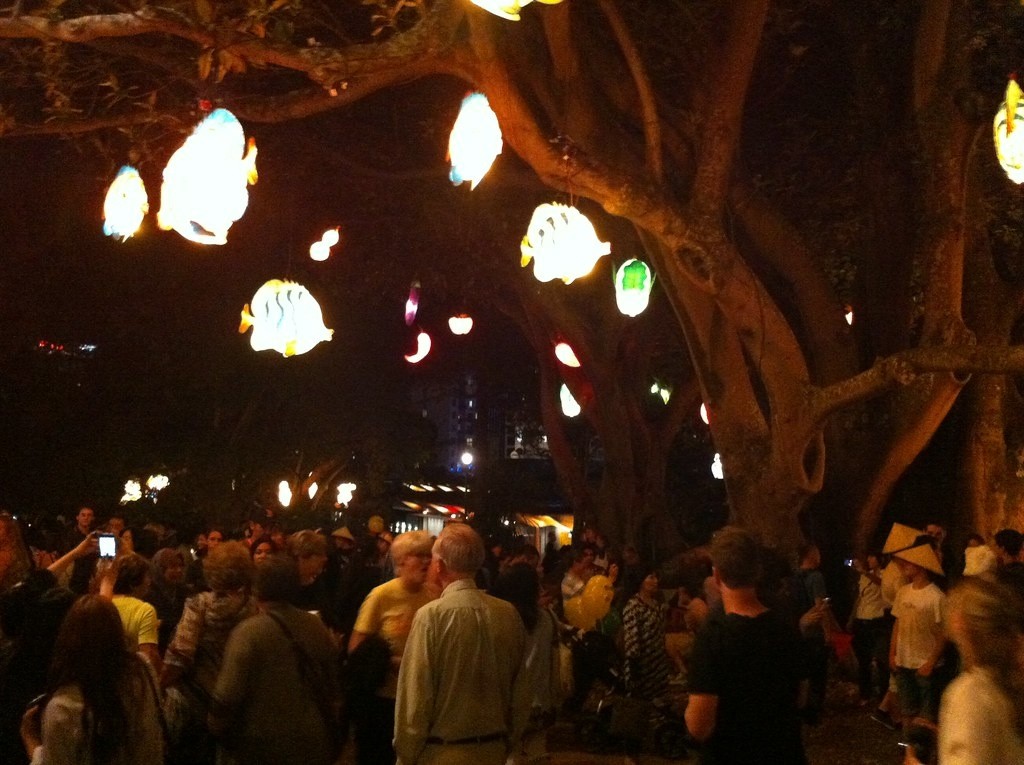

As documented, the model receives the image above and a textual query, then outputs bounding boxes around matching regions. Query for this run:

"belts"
[425,731,506,746]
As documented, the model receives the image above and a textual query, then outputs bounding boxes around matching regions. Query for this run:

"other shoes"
[870,708,896,732]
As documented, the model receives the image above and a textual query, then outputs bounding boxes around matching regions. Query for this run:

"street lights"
[462,452,472,523]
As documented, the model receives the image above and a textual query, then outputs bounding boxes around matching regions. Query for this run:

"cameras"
[898,724,935,754]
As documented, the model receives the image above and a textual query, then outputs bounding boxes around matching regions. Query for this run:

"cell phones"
[97,533,117,558]
[844,560,853,567]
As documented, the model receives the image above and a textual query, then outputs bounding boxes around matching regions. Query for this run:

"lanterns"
[100,1,724,481]
[991,80,1024,185]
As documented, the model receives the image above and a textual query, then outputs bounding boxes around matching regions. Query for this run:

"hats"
[880,522,928,554]
[329,526,354,539]
[894,544,944,575]
[378,530,394,543]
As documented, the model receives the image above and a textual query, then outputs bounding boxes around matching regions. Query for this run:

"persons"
[0,504,1024,765]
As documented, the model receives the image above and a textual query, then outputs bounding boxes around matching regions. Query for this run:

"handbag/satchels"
[163,719,217,765]
[310,687,345,752]
[580,677,649,740]
